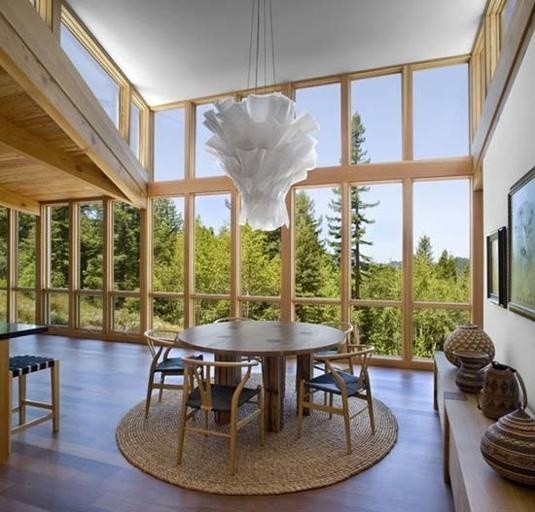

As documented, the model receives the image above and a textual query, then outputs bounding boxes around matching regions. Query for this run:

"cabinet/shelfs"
[429,345,535,511]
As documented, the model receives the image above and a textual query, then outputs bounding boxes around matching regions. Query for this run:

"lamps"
[198,0,322,238]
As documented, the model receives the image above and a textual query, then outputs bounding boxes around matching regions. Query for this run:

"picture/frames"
[484,225,508,310]
[503,165,535,324]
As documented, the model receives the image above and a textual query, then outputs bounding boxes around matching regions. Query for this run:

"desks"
[0,318,52,472]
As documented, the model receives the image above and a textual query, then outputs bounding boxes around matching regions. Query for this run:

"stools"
[9,352,59,450]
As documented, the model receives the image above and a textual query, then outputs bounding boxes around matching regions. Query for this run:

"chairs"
[137,316,377,480]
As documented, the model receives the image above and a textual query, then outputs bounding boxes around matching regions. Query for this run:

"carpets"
[114,370,400,499]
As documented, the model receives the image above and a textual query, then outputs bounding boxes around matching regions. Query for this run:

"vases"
[448,347,493,396]
